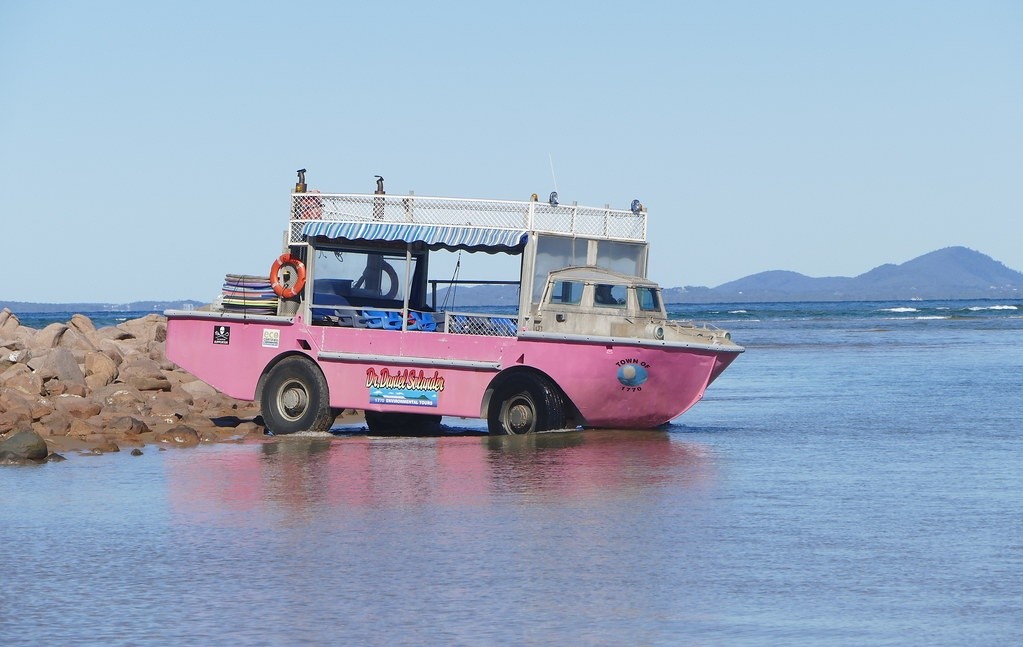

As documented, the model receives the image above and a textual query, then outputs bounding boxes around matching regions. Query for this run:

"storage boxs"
[314,279,353,293]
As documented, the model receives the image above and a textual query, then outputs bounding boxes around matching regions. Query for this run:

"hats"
[598,284,613,288]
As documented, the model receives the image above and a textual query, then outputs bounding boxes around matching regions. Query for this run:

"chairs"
[313,292,518,336]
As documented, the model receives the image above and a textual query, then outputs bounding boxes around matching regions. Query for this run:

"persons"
[596,285,617,304]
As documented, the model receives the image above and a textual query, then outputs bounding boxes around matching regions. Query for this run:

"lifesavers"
[270,254,306,299]
[368,260,399,299]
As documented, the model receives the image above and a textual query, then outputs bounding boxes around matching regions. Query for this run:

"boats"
[163,168,745,434]
